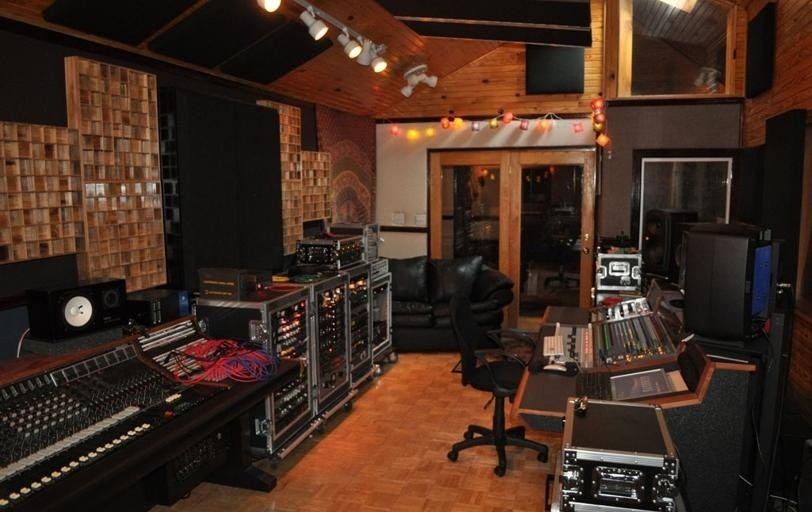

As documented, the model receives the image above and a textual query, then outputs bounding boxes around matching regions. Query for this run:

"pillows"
[379,256,431,304]
[427,254,483,305]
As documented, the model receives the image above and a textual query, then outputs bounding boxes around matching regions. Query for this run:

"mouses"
[543,364,567,372]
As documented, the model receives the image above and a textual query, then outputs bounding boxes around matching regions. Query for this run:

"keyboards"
[542,335,564,356]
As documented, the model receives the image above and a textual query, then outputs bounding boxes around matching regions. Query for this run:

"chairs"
[543,235,579,291]
[448,291,549,476]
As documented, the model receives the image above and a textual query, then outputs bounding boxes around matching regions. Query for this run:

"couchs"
[378,254,515,352]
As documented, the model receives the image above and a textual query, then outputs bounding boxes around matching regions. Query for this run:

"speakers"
[683,221,775,348]
[26,276,130,342]
[641,208,688,288]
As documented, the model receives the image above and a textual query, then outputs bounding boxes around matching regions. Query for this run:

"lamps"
[299,10,329,41]
[371,47,388,74]
[357,40,373,65]
[337,33,363,59]
[400,64,438,98]
[255,0,282,13]
[386,97,615,147]
